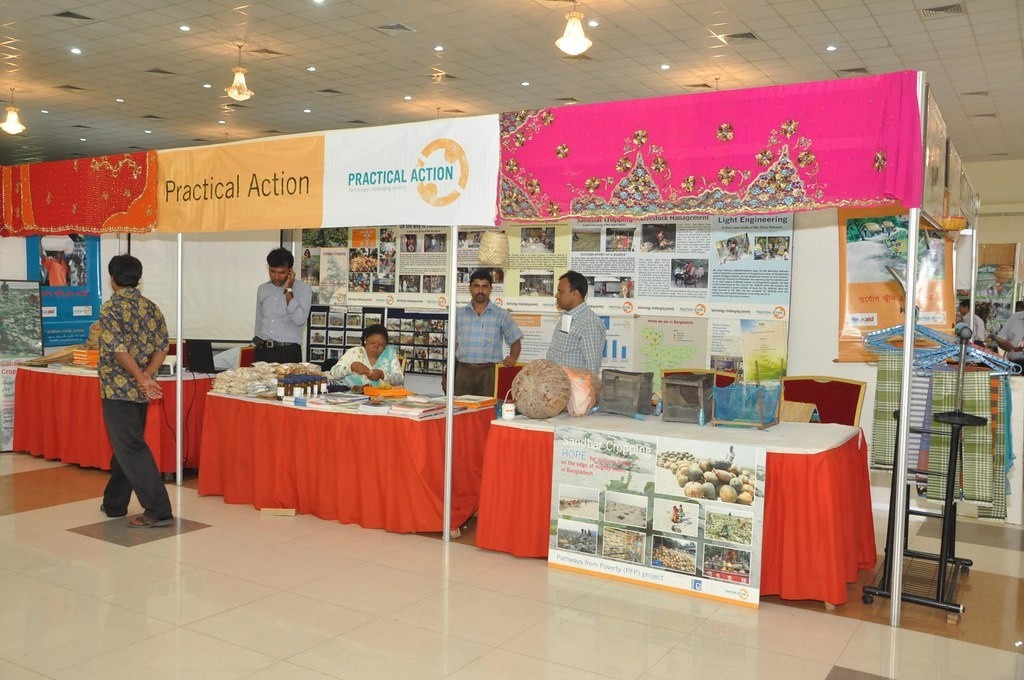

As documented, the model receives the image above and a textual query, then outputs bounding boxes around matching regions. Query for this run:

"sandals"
[127,516,173,528]
[100,504,111,518]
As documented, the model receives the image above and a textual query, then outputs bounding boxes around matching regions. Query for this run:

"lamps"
[555,1,593,57]
[0,82,27,133]
[225,42,256,102]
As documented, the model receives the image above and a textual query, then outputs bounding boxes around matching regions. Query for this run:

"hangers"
[859,305,1022,383]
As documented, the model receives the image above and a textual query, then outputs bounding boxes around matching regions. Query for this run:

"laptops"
[185,339,229,374]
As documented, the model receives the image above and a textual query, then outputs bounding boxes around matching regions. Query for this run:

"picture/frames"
[311,311,448,374]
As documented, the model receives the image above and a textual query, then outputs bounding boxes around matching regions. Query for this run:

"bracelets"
[283,287,292,294]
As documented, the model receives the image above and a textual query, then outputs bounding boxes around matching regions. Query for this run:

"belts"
[264,340,297,349]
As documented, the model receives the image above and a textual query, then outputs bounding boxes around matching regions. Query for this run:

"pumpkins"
[559,539,588,552]
[656,450,754,506]
[511,359,570,418]
[652,545,694,573]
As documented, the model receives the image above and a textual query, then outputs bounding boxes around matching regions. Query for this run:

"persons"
[995,311,1024,376]
[725,446,736,464]
[303,249,311,279]
[98,255,175,530]
[726,237,789,260]
[456,223,705,300]
[630,533,643,563]
[960,298,988,367]
[331,324,404,389]
[251,248,312,363]
[349,226,444,293]
[727,549,737,564]
[671,504,684,522]
[442,270,523,395]
[547,271,605,371]
[39,246,69,287]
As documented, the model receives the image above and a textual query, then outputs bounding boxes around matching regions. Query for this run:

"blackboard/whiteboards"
[127,230,284,344]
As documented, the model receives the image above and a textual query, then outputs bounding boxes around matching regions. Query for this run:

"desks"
[12,371,214,480]
[478,411,877,611]
[196,372,501,534]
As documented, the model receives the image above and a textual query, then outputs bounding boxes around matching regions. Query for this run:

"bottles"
[502,401,515,420]
[276,374,327,401]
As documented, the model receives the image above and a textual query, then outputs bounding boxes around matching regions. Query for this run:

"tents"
[0,71,982,625]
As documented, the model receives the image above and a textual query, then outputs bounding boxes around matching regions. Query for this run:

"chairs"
[238,345,254,367]
[660,369,739,421]
[493,364,533,404]
[781,377,866,425]
[169,338,188,368]
